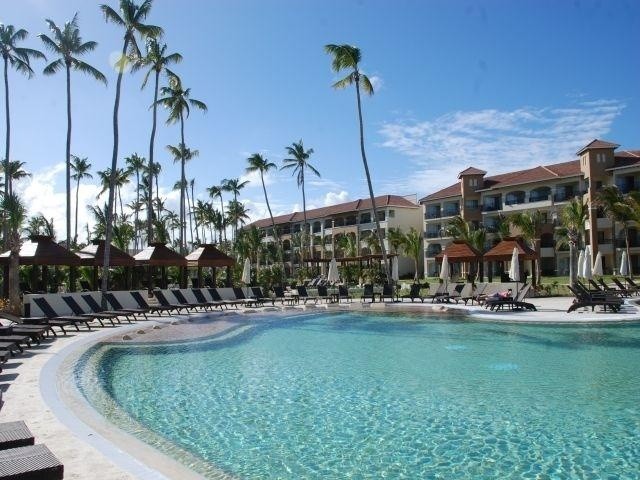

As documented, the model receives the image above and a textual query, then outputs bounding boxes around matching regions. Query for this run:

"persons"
[488,289,512,298]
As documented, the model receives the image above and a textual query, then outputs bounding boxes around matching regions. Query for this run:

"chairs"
[567,278,640,313]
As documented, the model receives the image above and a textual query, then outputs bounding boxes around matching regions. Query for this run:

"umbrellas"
[328,258,340,301]
[440,254,451,302]
[241,256,251,298]
[508,245,521,308]
[578,245,628,311]
